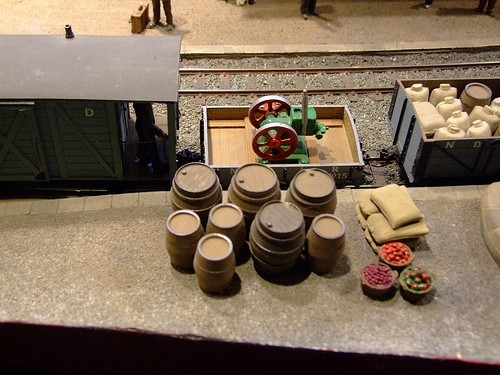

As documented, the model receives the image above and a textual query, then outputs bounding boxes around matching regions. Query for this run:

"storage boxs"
[386,74,500,184]
[199,100,367,180]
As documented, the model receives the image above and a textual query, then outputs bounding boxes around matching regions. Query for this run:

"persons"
[424,0,433,9]
[147,0,175,33]
[474,0,497,17]
[134,102,160,173]
[300,0,320,20]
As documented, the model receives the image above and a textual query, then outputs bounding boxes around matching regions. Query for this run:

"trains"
[0,23,499,195]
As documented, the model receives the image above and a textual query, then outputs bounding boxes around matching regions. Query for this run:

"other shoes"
[147,20,159,28]
[167,23,173,32]
[486,9,494,17]
[474,8,483,12]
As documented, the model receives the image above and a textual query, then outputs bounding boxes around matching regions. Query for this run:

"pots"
[395,265,438,294]
[378,241,416,268]
[361,263,398,291]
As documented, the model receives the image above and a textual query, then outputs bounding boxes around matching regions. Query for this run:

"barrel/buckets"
[304,214,346,276]
[205,203,246,262]
[460,82,492,114]
[226,162,281,236]
[164,209,204,273]
[193,233,235,294]
[285,168,337,235]
[249,200,304,273]
[170,162,223,229]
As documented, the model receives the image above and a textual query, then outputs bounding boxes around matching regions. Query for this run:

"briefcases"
[129,3,151,34]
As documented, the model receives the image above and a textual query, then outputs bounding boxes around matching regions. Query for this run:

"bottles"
[405,82,500,139]
